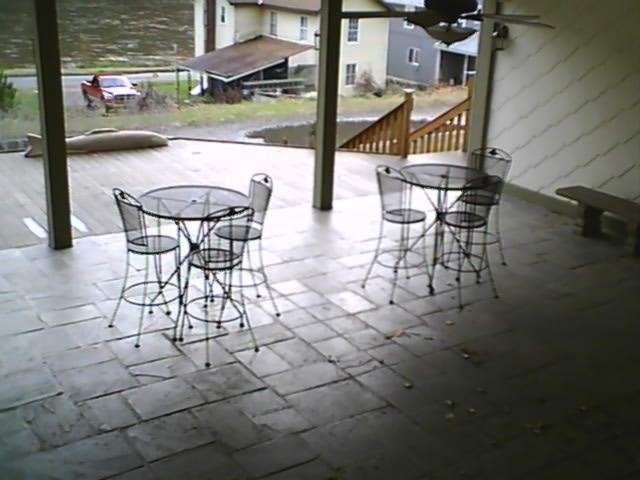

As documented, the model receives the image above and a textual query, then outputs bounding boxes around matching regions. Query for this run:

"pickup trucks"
[81,72,143,115]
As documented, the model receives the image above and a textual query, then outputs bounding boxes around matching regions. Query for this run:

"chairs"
[107,171,279,369]
[362,147,513,310]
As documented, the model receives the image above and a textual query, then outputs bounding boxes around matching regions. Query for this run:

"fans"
[330,0,557,47]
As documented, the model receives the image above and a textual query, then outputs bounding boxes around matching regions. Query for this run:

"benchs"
[554,185,639,240]
